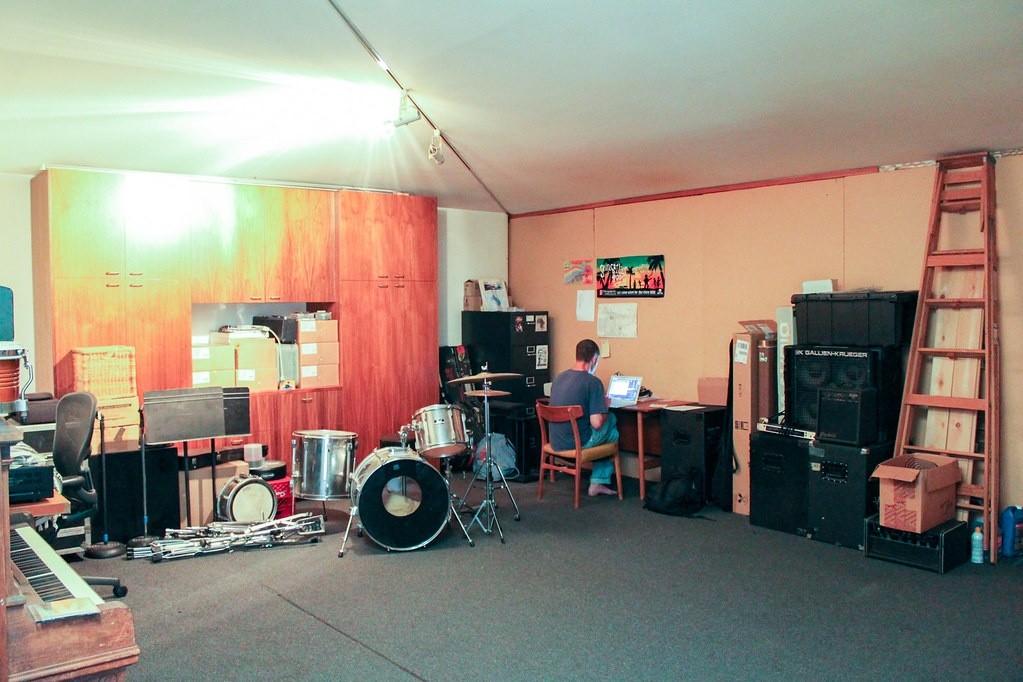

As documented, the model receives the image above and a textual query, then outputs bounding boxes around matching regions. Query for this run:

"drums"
[413,404,470,457]
[217,474,277,525]
[292,428,357,501]
[350,445,454,551]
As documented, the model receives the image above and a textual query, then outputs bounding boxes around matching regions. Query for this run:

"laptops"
[606,375,642,408]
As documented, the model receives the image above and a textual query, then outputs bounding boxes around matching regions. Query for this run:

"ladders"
[895,153,996,561]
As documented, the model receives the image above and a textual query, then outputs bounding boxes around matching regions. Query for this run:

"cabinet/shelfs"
[336,190,440,473]
[191,181,336,302]
[277,387,342,475]
[463,311,550,401]
[191,388,277,462]
[31,169,191,458]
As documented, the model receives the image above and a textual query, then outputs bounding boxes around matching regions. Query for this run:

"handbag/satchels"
[643,475,701,517]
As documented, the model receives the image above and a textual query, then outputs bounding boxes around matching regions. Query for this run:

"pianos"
[1,412,142,682]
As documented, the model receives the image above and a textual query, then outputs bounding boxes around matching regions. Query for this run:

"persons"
[548,339,619,496]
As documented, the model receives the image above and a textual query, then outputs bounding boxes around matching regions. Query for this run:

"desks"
[537,398,698,500]
[10,488,70,516]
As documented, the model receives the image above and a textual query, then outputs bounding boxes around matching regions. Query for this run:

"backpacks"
[472,434,520,481]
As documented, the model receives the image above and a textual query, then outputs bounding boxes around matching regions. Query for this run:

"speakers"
[749,345,897,552]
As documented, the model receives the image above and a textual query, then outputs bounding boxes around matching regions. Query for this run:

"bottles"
[971,526,984,564]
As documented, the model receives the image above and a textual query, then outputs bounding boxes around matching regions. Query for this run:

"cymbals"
[447,371,524,383]
[464,389,511,396]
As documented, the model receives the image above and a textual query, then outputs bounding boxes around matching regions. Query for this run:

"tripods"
[456,379,521,542]
[127,512,325,563]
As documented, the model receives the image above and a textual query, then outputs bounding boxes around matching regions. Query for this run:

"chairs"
[536,401,623,508]
[29,392,128,598]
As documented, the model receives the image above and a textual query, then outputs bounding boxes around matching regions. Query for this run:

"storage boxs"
[870,452,963,533]
[267,476,296,519]
[464,279,512,312]
[733,307,797,516]
[88,397,139,456]
[8,466,55,504]
[191,316,339,392]
[178,449,248,529]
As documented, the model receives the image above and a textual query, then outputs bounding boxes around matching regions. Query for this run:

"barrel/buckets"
[1001,505,1023,557]
